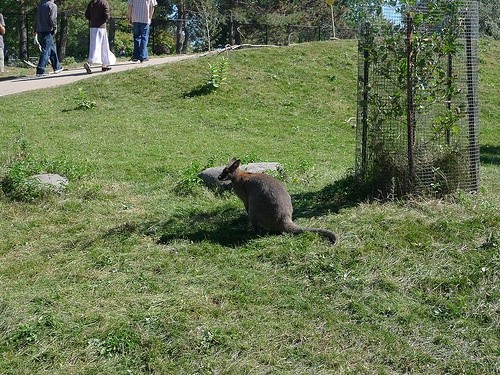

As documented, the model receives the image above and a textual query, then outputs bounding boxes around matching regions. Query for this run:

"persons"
[32,0,63,77]
[0,13,6,72]
[127,0,158,62]
[84,0,117,74]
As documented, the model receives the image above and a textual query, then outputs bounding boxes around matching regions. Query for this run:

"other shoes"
[141,59,149,63]
[84,63,93,74]
[102,66,112,71]
[130,58,139,63]
[53,66,65,73]
[36,73,49,76]
